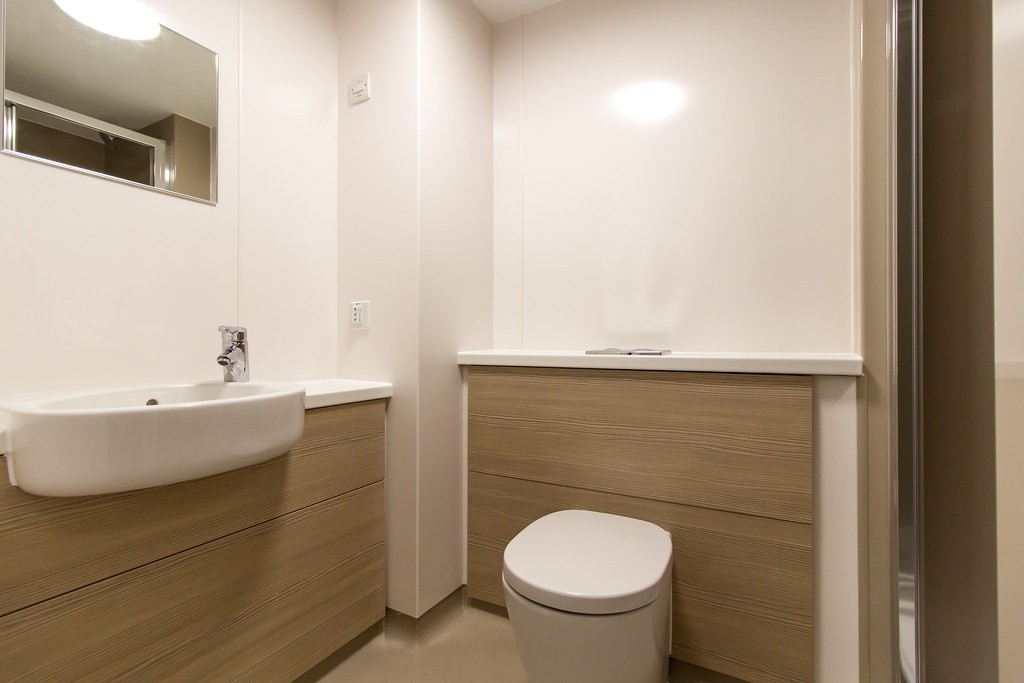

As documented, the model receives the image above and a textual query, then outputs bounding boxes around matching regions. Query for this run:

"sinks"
[5,382,306,496]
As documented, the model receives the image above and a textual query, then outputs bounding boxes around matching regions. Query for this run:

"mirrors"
[0,0,218,206]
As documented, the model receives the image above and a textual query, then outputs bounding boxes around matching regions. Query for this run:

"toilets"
[500,510,672,682]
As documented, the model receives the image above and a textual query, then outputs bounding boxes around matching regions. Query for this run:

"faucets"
[216,325,249,382]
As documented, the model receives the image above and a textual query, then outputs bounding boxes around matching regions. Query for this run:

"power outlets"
[349,300,371,331]
